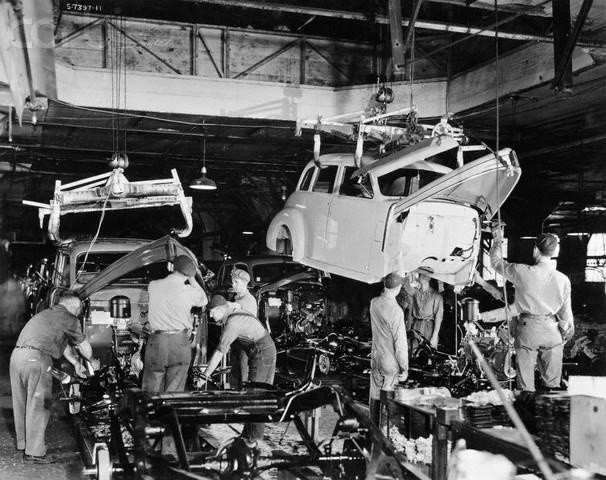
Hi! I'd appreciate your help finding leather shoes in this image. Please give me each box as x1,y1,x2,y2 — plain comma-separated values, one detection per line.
23,454,58,464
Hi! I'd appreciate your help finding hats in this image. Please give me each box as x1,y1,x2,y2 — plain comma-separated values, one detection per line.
175,255,196,277
205,295,228,309
536,232,557,251
385,273,401,289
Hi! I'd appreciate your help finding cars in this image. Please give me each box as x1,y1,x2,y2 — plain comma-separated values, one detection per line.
11,234,209,381
210,252,322,337
262,130,524,296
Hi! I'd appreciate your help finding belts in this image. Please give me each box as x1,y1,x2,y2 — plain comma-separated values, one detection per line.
520,313,555,318
155,330,188,334
16,346,39,351
414,318,433,322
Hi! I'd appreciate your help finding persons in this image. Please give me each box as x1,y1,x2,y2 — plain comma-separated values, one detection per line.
368,272,409,430
140,253,209,393
8,290,101,463
489,225,576,391
405,265,445,350
197,293,279,449
230,268,259,387
